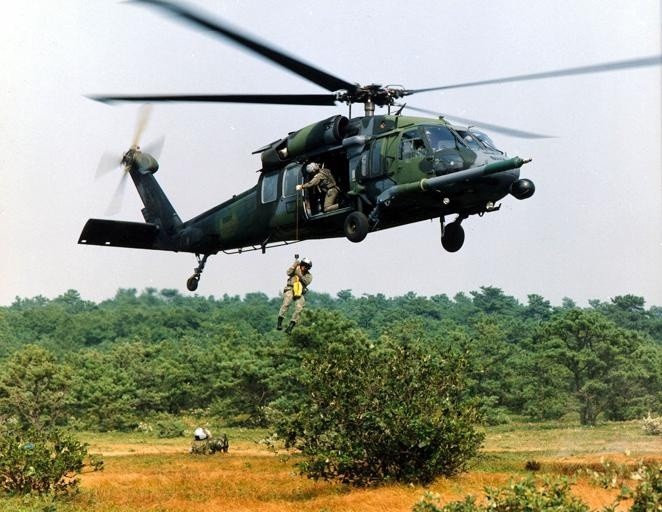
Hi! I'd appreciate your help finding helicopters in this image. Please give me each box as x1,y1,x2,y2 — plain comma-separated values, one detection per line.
78,1,662,292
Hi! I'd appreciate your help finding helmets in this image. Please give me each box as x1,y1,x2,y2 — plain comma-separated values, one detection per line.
300,257,312,269
306,162,319,173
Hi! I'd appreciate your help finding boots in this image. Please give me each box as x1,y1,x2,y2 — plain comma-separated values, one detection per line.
277,316,284,330
285,321,296,336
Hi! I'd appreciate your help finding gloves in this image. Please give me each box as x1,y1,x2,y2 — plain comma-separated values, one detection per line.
295,184,303,191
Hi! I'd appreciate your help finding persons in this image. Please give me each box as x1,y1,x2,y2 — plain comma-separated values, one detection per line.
277,257,314,336
296,162,345,212
